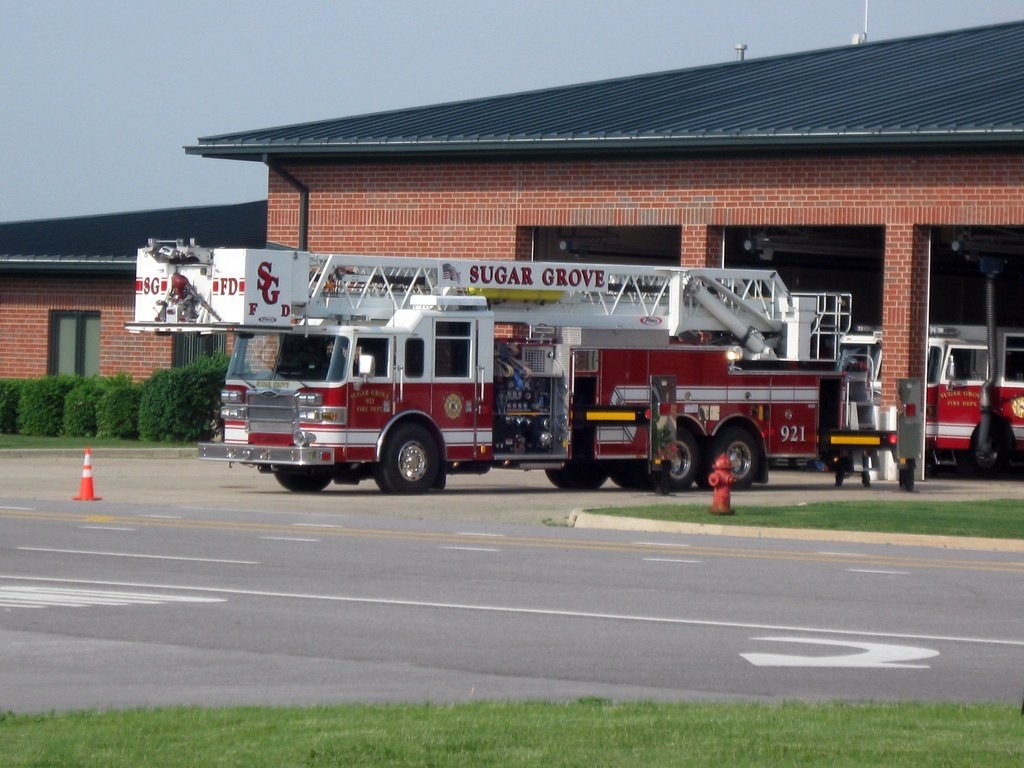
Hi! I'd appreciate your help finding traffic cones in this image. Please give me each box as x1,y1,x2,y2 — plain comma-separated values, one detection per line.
69,448,105,500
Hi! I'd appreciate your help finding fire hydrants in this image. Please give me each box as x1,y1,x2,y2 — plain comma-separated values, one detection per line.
708,450,737,514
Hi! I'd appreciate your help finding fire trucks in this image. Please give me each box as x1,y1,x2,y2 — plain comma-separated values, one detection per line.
833,323,1024,479
122,232,894,494
